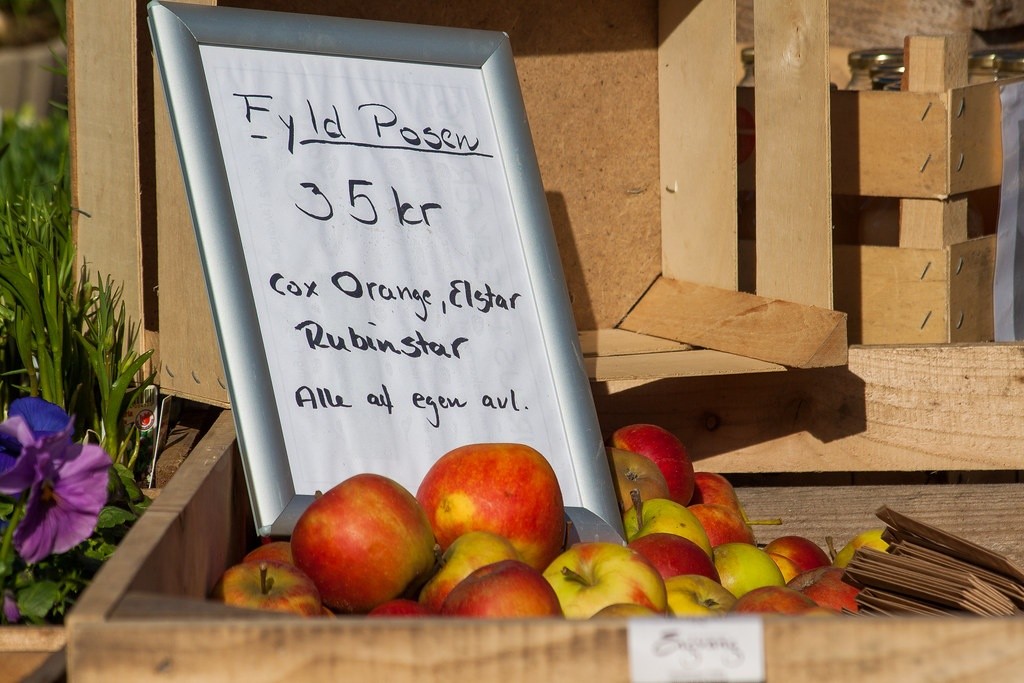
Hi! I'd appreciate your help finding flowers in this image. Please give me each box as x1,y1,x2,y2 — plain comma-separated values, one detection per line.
0,149,164,622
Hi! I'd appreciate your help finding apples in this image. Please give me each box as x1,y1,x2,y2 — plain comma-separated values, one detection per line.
205,424,893,618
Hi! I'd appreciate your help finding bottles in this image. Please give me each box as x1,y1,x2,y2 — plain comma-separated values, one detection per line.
739,46,1024,93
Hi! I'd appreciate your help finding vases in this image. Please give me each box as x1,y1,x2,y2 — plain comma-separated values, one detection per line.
0,382,239,683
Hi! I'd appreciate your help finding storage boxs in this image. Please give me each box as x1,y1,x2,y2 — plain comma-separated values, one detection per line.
64,341,1024,683
